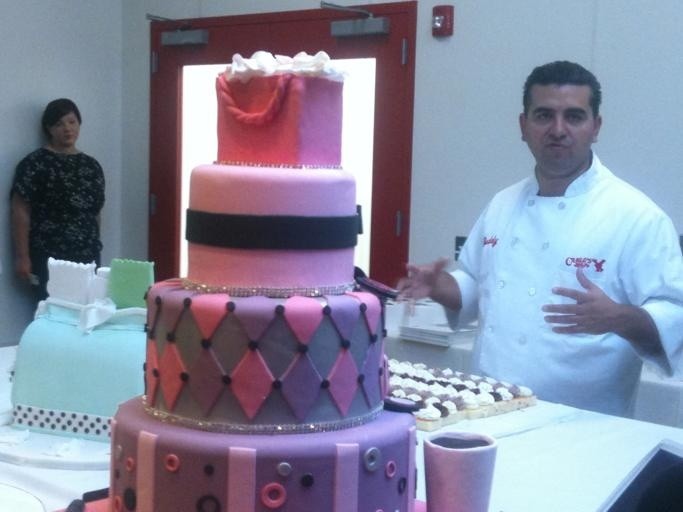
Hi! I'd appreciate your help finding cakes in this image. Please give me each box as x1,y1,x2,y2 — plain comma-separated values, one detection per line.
388,356,538,432
0,258,154,467
67,51,496,512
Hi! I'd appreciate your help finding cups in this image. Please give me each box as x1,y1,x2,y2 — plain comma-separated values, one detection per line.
423,432,497,511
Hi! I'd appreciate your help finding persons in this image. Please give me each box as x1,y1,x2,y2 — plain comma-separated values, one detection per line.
10,98,106,302
396,61,683,420
